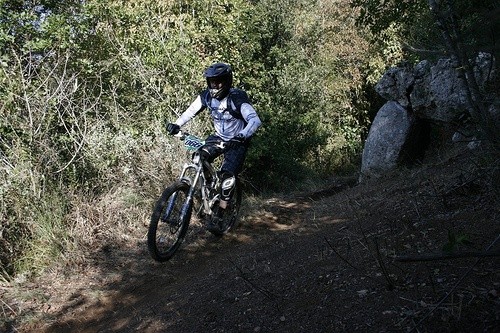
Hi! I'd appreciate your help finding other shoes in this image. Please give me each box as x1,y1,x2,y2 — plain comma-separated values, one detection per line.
206,216,224,231
197,183,210,198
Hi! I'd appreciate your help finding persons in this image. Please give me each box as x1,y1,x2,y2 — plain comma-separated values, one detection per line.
166,62,261,218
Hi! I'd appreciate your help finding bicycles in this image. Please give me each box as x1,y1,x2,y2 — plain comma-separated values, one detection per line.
147,124,243,263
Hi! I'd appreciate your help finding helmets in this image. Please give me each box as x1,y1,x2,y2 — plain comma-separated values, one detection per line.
202,63,233,98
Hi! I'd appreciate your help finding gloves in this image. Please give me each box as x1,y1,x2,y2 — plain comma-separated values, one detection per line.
165,123,180,136
228,135,243,150
